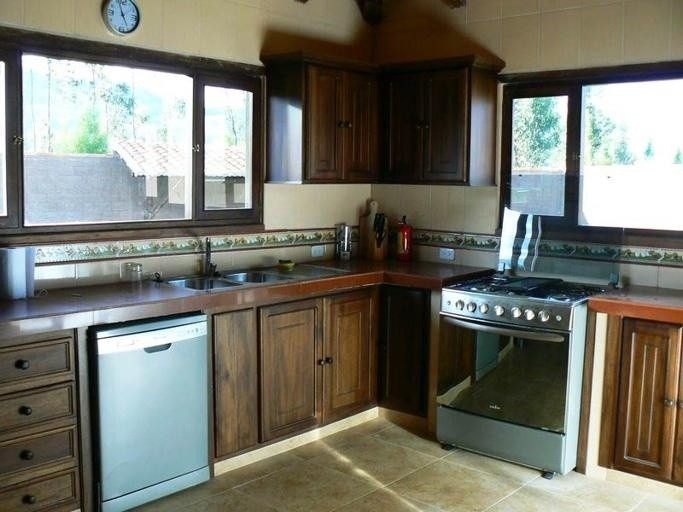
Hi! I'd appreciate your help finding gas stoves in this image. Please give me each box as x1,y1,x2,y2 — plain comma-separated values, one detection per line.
440,269,601,329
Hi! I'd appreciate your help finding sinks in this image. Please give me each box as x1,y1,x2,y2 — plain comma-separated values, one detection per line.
162,274,241,290
223,270,296,283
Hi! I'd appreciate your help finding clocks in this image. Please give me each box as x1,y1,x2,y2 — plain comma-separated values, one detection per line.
101,0,140,37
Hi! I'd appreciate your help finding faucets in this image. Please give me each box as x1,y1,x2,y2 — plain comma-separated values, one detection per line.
205,236,218,279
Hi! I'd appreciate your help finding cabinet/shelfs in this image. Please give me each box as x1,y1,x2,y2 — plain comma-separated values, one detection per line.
256,286,378,445
378,283,431,419
258,50,505,188
597,317,682,488
0,328,83,512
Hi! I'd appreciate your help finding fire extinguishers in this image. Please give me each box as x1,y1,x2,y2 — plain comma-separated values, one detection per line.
397,215,411,262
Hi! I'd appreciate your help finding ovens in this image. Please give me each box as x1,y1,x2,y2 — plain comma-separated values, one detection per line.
435,310,581,478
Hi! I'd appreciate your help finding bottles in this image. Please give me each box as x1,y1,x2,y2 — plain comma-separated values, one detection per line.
336,224,352,263
126,263,141,287
